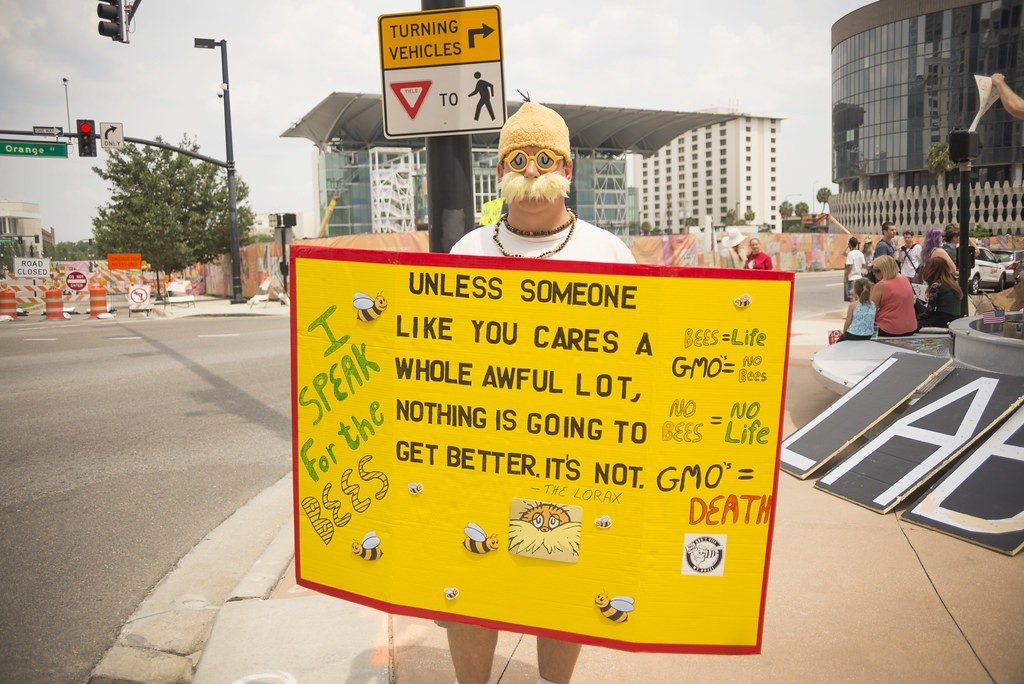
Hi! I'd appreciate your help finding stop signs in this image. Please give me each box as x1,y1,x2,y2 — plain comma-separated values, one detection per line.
65,272,87,290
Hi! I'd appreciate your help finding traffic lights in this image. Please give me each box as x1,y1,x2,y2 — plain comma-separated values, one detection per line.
195,38,248,303
283,214,296,227
269,214,281,228
77,120,96,157
97,0,127,43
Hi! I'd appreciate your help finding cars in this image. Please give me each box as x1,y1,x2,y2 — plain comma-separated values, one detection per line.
969,247,1006,294
989,249,1024,288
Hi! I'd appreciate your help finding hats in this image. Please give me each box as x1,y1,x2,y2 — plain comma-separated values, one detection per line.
497,89,570,163
722,229,747,247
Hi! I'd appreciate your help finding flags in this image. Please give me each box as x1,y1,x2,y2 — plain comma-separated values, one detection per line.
983,310,1005,324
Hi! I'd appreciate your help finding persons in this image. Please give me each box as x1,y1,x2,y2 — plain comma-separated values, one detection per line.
917,256,963,328
862,238,874,277
873,222,896,260
990,72,1024,120
836,279,876,342
870,255,918,336
921,228,956,274
893,229,922,284
435,102,637,684
941,223,960,268
744,238,773,270
844,237,866,319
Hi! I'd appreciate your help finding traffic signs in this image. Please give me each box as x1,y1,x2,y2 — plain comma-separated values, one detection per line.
33,126,64,137
378,4,506,140
101,122,124,149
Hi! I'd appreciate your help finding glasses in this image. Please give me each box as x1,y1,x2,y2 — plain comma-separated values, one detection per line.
504,149,564,172
873,269,880,273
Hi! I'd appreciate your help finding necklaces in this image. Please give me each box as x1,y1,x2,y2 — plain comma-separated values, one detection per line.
492,208,578,259
